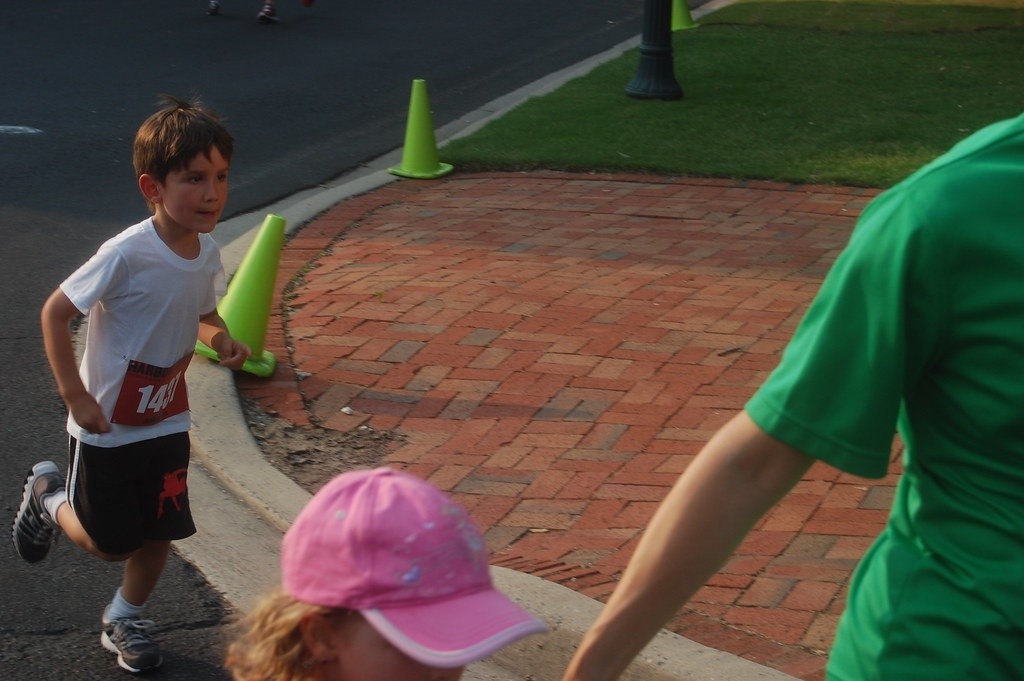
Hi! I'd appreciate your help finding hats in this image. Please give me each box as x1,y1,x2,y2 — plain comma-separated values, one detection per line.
282,465,548,668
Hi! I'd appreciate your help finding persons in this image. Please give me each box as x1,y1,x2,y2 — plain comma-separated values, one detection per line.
560,111,1024,681
12,94,252,672
226,466,549,681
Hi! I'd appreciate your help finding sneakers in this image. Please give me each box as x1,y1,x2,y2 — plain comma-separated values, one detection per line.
101,603,162,672
11,460,65,565
